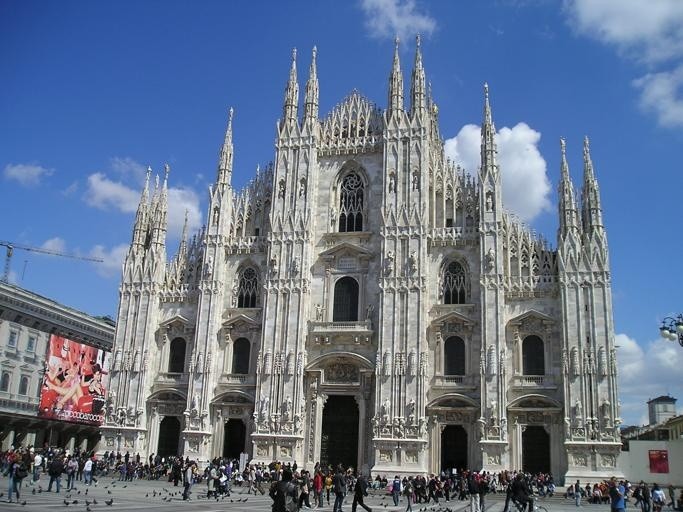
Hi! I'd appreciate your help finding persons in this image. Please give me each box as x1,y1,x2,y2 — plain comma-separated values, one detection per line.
43,363,107,413
562,476,683,512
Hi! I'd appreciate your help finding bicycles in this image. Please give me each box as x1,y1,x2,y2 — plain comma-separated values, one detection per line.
506,493,550,512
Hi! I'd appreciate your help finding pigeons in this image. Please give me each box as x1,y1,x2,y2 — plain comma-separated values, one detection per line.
0,481,127,512
343,494,467,512
145,488,249,503
